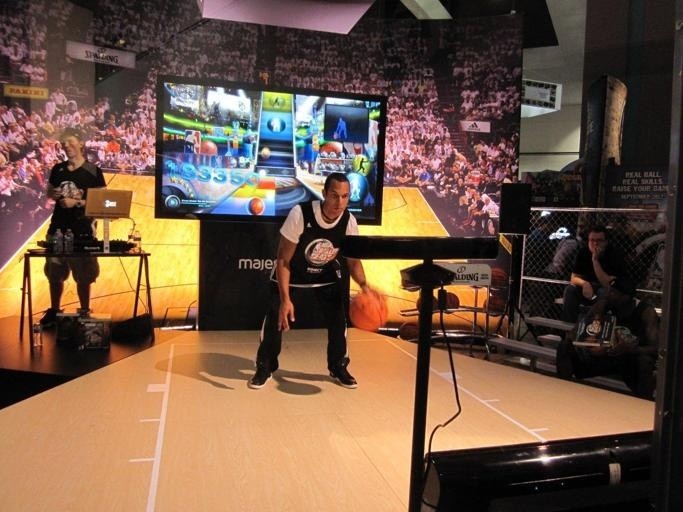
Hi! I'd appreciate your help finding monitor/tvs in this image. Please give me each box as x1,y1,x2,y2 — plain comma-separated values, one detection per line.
154,75,388,226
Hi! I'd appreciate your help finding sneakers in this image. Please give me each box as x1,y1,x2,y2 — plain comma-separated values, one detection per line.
39,308,63,327
247,368,273,390
328,366,359,390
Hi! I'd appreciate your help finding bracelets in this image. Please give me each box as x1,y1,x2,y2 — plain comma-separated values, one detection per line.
358,281,366,288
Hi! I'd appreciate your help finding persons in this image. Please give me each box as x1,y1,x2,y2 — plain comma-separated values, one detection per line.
518,208,662,402
39,127,107,326
1,0,526,239
246,171,387,389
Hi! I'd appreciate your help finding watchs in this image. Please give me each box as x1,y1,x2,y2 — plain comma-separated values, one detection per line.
75,198,80,208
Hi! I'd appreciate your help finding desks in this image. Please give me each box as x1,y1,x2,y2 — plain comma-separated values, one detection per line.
17,246,155,344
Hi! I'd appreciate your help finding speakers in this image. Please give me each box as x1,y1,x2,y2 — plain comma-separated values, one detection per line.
500,182,531,236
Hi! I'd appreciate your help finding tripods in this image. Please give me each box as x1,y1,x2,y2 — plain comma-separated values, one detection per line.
484,235,543,360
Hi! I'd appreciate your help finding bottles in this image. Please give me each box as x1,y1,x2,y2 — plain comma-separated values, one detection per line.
127,228,133,252
46,229,73,254
133,230,141,253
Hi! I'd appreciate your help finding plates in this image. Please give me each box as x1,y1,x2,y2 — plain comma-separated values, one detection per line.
26,248,48,254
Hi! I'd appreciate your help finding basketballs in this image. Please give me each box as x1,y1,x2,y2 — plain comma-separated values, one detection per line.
416,296,438,313
483,296,505,317
492,267,507,281
399,321,419,340
350,291,389,330
444,293,459,314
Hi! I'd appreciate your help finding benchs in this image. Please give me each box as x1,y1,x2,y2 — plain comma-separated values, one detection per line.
485,294,662,399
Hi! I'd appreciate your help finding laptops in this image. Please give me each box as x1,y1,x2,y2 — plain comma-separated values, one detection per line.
85,188,133,218
